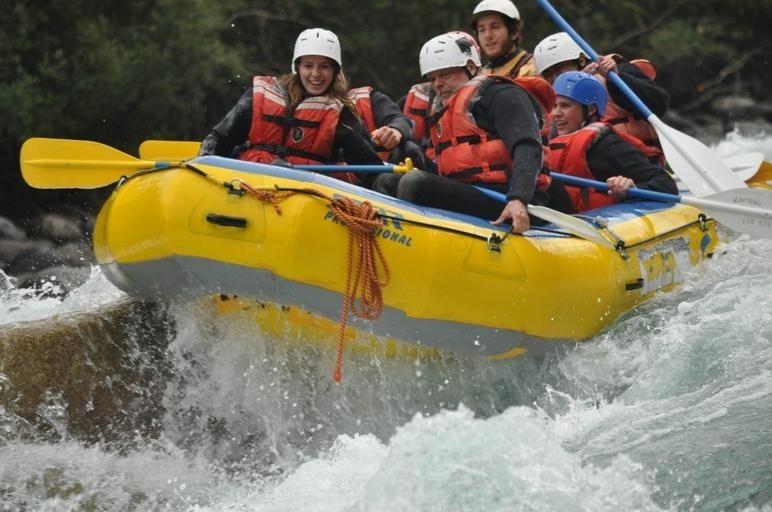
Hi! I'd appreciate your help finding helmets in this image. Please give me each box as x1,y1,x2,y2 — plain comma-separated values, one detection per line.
471,0,521,24
533,32,592,77
291,27,342,73
418,32,481,78
552,70,608,118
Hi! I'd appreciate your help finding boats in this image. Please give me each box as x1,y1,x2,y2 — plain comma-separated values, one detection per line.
91,162,772,359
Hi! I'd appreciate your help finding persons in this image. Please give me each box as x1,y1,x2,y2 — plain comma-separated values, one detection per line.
197,29,388,188
323,0,678,234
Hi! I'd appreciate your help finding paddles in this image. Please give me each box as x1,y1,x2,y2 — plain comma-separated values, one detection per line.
472,185,609,246
552,173,772,219
666,152,763,192
19,137,414,190
539,0,749,198
135,140,204,162
